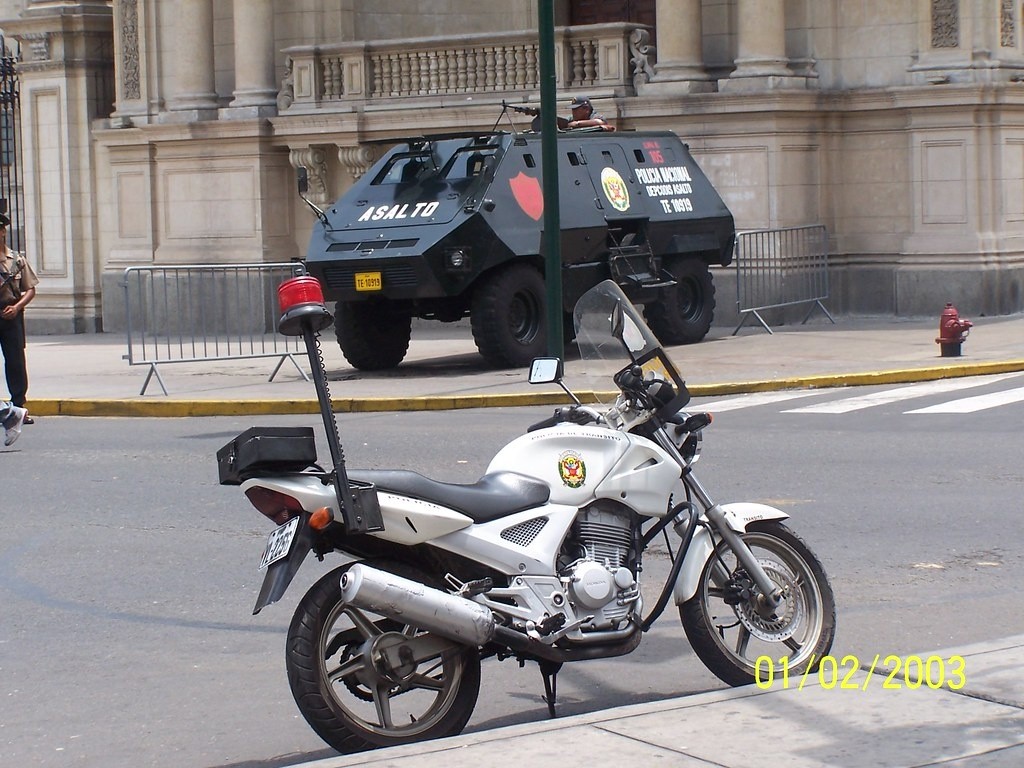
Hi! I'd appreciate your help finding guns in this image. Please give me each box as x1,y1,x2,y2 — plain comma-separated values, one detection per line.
493,100,568,136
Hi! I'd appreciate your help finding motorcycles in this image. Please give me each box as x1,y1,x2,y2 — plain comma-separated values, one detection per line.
216,264,837,757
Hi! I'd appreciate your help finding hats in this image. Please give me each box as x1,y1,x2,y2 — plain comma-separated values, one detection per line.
0,212,10,228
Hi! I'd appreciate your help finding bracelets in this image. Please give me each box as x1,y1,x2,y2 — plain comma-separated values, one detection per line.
576,120,579,128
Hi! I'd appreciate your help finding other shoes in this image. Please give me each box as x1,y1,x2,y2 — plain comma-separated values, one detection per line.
25,417,34,425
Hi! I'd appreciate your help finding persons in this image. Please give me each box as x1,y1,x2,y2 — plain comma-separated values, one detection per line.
0,212,40,424
0,399,28,447
567,95,607,129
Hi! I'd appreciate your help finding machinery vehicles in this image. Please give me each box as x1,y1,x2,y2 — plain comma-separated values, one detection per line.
295,100,736,368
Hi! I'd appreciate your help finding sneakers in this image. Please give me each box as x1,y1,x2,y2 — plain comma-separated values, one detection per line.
5,407,28,446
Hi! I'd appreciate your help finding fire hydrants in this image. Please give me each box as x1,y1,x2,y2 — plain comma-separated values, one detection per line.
935,302,973,358
